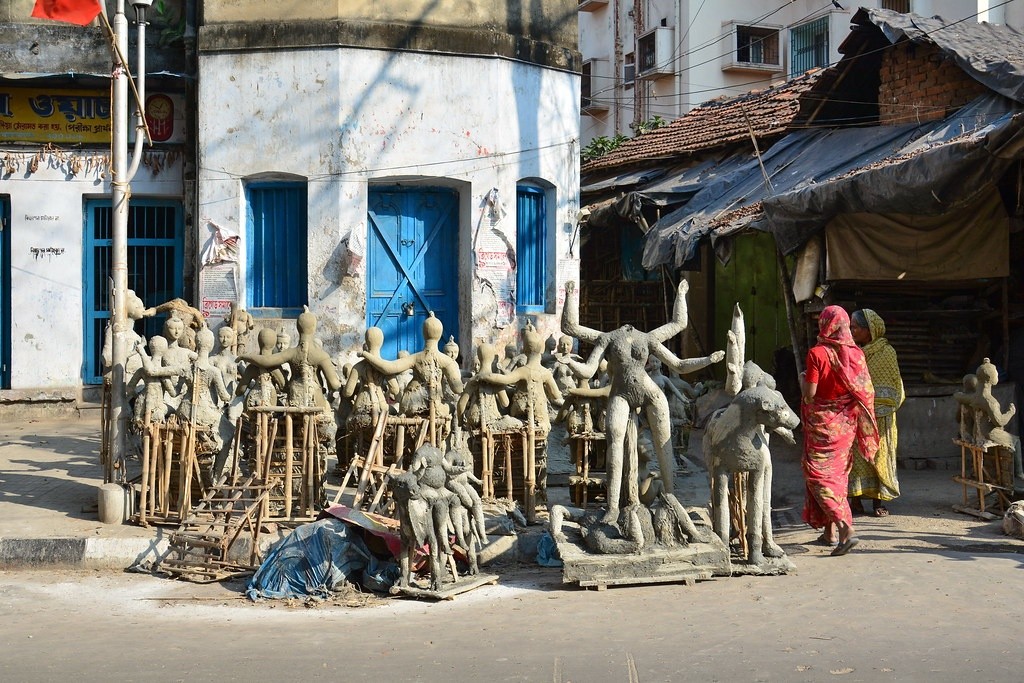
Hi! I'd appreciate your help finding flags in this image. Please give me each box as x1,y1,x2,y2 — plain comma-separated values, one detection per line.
31,0,102,29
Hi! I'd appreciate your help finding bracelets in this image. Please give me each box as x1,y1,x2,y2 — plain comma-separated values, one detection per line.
799,372,806,376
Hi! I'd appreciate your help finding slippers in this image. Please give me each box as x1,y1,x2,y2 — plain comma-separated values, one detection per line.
817,534,840,546
831,537,859,556
850,506,865,514
872,506,889,516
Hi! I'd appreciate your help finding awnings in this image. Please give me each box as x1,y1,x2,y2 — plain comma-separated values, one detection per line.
581,145,769,352
641,91,1023,373
581,165,678,194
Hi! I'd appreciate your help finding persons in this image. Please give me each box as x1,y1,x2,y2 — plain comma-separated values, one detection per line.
798,305,880,555
953,359,1024,480
98,285,800,565
847,309,906,517
